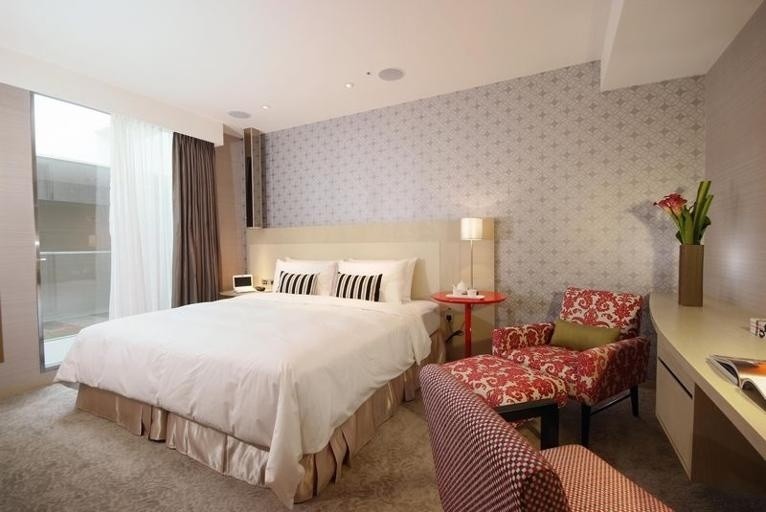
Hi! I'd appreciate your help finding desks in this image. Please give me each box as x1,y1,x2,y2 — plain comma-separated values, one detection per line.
431,290,507,358
648,294,766,482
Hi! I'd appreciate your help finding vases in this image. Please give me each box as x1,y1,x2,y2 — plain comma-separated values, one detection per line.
678,245,704,307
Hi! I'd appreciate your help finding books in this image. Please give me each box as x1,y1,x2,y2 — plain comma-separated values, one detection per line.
750,318,766,340
706,354,766,402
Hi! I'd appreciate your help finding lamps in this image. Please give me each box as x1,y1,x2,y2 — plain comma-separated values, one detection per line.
461,217,483,290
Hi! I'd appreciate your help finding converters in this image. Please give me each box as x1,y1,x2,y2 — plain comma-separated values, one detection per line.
447,315,451,320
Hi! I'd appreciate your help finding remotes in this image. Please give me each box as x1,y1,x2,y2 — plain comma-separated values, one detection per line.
255,287,265,291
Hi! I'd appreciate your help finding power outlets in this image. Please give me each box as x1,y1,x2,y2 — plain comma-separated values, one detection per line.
444,311,453,322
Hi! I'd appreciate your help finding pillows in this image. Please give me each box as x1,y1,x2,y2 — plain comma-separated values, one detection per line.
549,320,620,351
271,256,418,304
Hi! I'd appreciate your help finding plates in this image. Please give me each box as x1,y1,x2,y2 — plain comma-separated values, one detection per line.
446,294,485,299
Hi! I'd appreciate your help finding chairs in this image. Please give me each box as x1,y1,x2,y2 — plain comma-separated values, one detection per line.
491,288,652,449
419,364,673,512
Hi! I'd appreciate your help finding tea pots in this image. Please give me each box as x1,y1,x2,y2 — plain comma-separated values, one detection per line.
454,281,467,295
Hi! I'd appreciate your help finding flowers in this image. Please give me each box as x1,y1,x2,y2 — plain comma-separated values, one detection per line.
653,181,713,245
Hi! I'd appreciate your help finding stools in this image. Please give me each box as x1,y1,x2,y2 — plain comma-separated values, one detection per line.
441,353,569,450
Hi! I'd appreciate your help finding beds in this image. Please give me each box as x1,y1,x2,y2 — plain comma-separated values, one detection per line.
54,217,496,511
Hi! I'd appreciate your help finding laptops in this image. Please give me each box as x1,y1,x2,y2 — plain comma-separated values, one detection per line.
232,274,257,293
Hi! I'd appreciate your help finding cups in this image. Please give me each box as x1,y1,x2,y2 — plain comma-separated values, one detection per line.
467,290,478,297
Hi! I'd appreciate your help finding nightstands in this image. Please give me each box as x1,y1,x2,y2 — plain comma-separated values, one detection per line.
220,291,238,297
